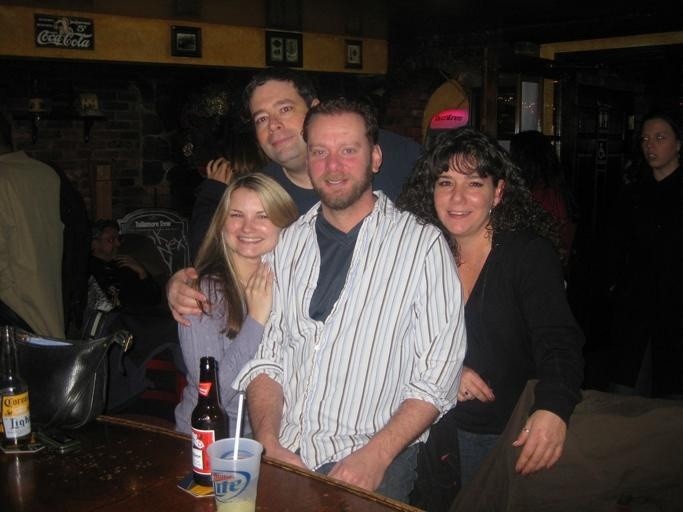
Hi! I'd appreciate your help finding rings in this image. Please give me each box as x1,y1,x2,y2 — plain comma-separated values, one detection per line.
521,429,530,432
463,390,469,396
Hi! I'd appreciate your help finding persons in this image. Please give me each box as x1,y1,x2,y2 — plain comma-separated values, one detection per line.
0,114,64,339
396,127,584,508
185,140,261,260
230,96,468,505
509,130,569,289
86,215,189,375
608,115,682,397
166,68,420,327
174,171,300,441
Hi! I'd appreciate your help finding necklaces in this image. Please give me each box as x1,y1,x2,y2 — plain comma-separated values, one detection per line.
458,249,491,272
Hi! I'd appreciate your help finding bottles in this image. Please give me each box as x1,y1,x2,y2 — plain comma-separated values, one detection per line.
0,324,33,456
190,356,230,489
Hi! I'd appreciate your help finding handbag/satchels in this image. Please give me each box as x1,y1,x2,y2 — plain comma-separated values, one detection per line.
14,324,136,454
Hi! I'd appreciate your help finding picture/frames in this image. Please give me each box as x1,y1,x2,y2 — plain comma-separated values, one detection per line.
266,29,304,69
343,39,362,69
35,13,96,51
170,23,203,58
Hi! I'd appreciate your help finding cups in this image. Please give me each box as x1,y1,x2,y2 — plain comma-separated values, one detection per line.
206,437,265,511
